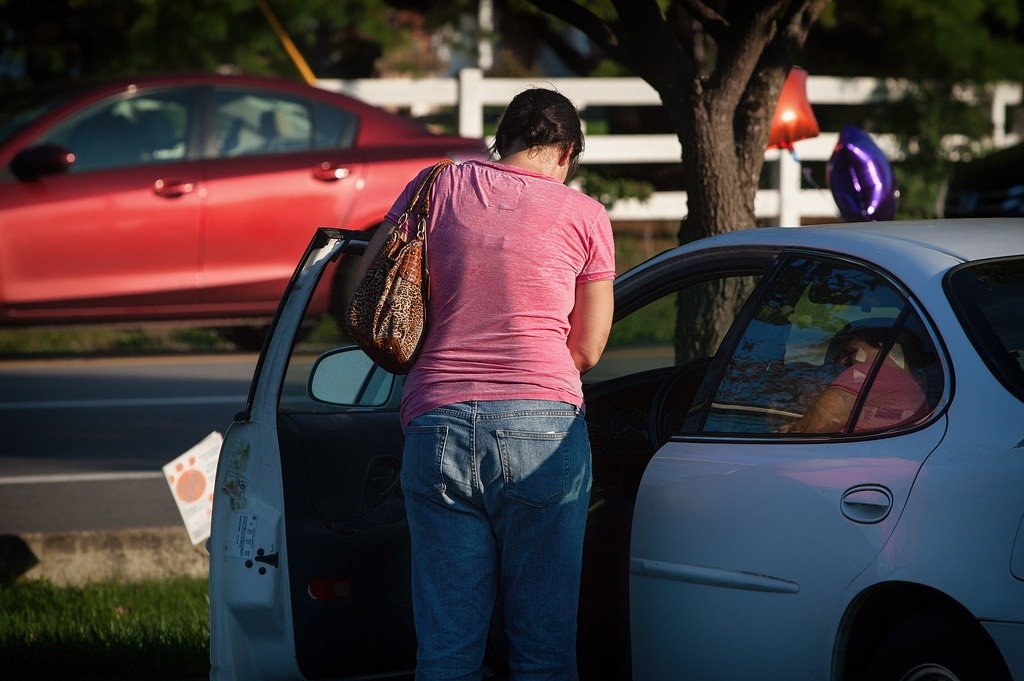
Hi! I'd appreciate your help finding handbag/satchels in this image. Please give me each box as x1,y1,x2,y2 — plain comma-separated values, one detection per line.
347,158,456,373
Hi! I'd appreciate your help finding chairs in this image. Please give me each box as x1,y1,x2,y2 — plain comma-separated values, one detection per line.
217,110,282,157
713,316,895,428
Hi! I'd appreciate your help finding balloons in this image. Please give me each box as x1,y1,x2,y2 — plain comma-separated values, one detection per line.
825,125,894,221
766,66,820,152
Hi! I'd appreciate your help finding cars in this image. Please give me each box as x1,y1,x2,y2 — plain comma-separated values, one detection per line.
208,223,1021,679
1,71,489,351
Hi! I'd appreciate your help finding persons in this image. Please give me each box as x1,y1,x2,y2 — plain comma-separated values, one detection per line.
776,329,931,433
344,89,615,680
